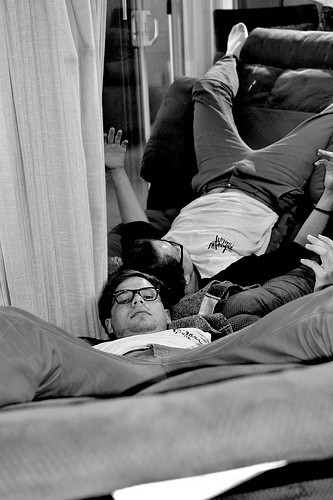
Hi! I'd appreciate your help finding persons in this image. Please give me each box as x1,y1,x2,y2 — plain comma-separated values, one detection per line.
0,234,333,406
103,22,333,288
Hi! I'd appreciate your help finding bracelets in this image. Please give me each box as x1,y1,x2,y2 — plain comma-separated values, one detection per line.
313,204,331,215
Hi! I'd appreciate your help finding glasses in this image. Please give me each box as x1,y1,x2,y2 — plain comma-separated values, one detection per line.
111,285,160,304
156,238,184,268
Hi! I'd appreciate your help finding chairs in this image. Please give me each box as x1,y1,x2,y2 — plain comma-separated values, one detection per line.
105,27,333,318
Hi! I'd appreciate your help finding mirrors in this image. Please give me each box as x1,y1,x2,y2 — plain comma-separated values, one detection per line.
102,0,333,326
